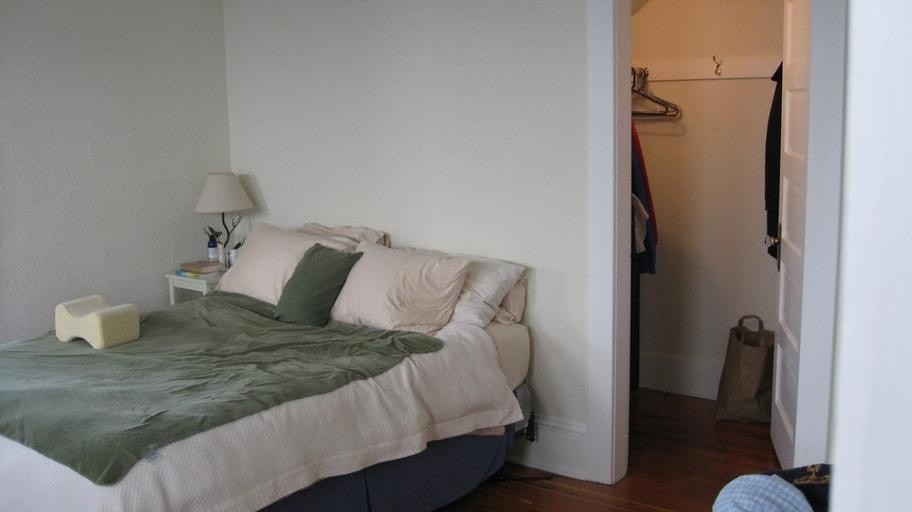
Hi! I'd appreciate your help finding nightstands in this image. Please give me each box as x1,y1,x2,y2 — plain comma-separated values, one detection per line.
164,270,226,305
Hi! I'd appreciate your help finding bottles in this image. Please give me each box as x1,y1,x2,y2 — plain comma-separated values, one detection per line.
223,249,229,269
217,240,225,267
229,249,237,267
206,233,218,261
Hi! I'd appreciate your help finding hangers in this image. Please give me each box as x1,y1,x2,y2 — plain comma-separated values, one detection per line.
630,65,680,120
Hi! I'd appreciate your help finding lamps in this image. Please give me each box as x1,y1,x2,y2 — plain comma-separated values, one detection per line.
192,171,257,269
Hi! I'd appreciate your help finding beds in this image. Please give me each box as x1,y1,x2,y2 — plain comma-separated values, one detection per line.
0,289,536,511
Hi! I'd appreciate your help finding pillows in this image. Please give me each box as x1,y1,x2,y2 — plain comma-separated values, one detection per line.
329,239,471,334
271,241,365,330
214,222,356,306
392,247,527,326
298,223,389,249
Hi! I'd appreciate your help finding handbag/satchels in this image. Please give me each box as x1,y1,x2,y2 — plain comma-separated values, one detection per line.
715,325,774,426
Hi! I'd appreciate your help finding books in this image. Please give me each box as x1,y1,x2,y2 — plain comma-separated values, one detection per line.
174,259,227,279
